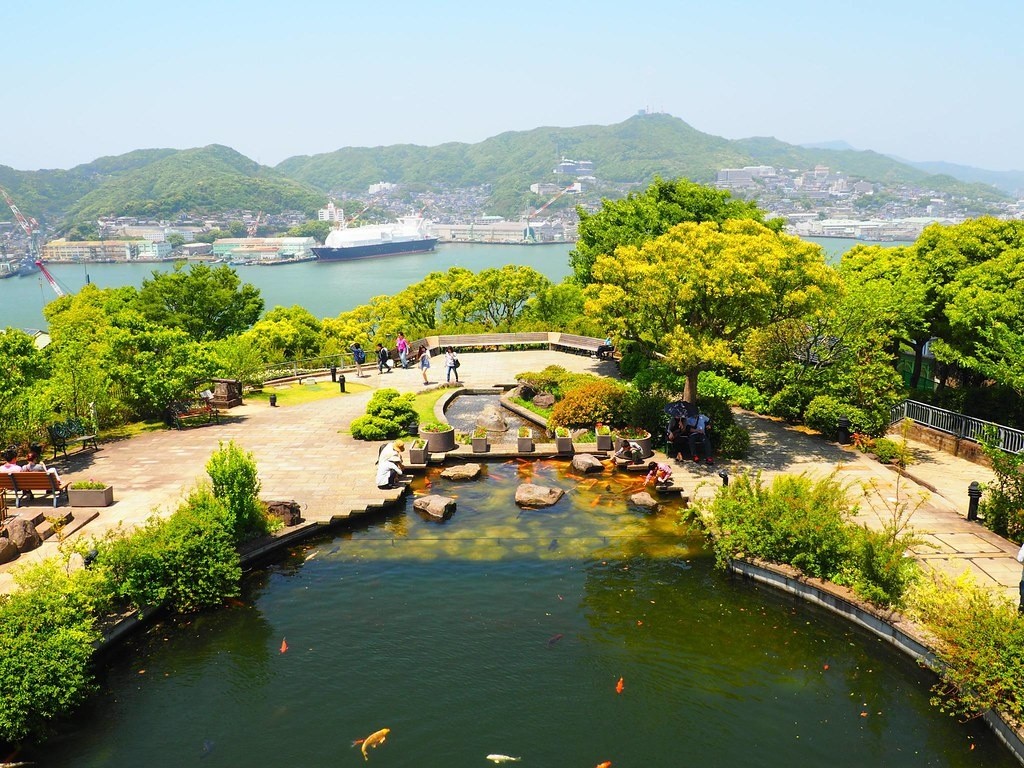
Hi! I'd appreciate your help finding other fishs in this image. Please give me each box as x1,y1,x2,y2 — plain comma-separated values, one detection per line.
587,469,655,507
547,635,562,645
279,637,288,653
327,546,341,556
412,477,462,498
306,549,319,561
521,507,538,511
230,598,244,607
590,495,601,506
351,728,390,761
486,754,522,764
566,473,583,481
201,741,218,760
0,751,36,768
548,539,558,552
615,675,624,693
488,474,503,480
501,458,566,484
596,761,611,768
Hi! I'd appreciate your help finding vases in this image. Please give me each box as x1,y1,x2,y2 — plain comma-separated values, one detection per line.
68,485,113,507
614,431,652,459
595,426,611,450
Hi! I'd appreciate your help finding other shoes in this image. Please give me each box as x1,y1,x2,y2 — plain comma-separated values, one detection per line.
378,371,382,374
403,366,405,369
628,461,634,465
456,379,458,382
355,373,359,376
424,381,428,385
387,367,391,372
361,375,364,377
678,454,684,462
46,490,52,494
676,455,679,461
693,455,699,462
406,364,407,369
707,457,713,463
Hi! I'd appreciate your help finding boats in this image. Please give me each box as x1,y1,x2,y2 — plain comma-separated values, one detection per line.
310,206,442,262
19,255,45,278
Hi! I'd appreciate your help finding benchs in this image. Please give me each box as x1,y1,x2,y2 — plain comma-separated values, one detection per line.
169,398,219,430
46,417,98,460
661,420,713,454
409,332,617,361
389,346,418,367
0,472,72,507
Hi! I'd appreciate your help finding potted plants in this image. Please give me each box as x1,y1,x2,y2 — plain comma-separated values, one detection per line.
555,426,571,452
419,422,454,452
472,426,488,452
409,438,428,463
517,425,532,452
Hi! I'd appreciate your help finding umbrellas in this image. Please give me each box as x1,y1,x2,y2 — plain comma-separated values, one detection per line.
664,400,698,417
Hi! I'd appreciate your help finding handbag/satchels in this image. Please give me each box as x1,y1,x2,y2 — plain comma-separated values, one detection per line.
454,359,460,368
681,425,697,436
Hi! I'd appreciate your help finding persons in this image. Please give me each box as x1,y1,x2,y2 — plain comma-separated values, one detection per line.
396,332,410,369
22,451,61,494
350,343,365,377
443,346,458,382
0,450,26,494
641,461,672,487
418,345,431,385
667,414,714,463
376,439,405,489
591,336,614,358
374,343,391,375
1017,543,1024,619
611,440,643,465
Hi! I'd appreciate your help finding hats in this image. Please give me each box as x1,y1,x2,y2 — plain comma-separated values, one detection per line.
393,439,405,452
388,456,401,461
687,408,698,416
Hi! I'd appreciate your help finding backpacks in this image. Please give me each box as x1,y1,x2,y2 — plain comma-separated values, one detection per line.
357,349,365,364
388,350,391,359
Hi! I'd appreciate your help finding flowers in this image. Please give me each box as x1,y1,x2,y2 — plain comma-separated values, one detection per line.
72,478,108,490
596,422,609,435
616,424,648,440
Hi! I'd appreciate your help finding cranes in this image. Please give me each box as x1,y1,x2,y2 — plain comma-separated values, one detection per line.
523,180,574,239
35,260,66,296
0,188,42,256
247,211,263,237
